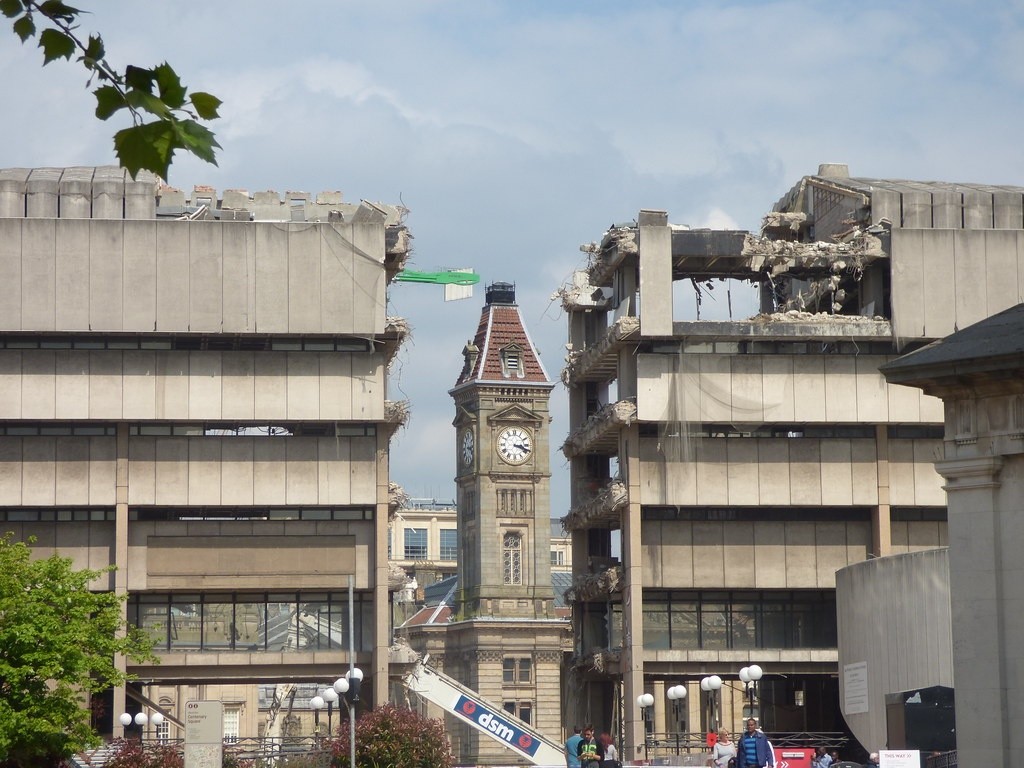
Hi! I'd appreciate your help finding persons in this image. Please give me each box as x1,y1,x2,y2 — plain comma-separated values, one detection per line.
564,725,619,768
829,750,842,768
736,719,778,768
811,746,833,768
864,753,879,768
713,727,737,768
707,729,717,751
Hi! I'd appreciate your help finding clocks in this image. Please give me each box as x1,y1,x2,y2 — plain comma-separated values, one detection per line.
497,426,533,463
462,432,473,465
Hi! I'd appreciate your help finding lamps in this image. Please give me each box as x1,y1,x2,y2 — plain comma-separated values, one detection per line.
196,197,213,206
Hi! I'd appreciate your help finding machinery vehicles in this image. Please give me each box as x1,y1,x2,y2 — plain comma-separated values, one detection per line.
258,612,573,768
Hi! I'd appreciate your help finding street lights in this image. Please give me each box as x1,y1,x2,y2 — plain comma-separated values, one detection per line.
151,713,163,744
334,667,364,768
309,696,324,749
700,674,721,749
120,713,148,744
667,684,687,757
323,688,339,737
638,693,653,761
739,665,763,720
